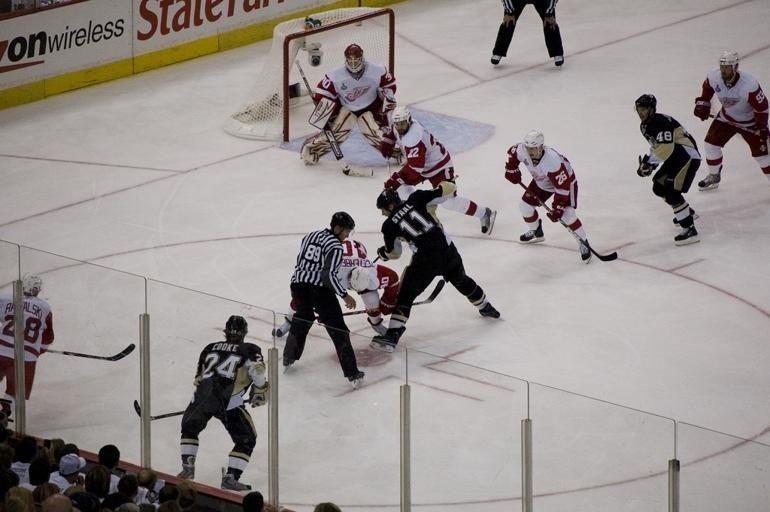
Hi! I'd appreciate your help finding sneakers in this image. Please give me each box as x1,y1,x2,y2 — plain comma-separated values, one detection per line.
277,317,296,364
349,370,364,382
491,55,502,64
673,167,723,241
479,304,500,318
177,456,195,479
481,208,491,233
520,219,543,241
221,467,251,490
580,239,590,260
555,56,564,66
367,318,406,345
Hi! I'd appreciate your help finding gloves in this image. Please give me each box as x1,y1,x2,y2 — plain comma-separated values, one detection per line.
378,246,389,261
694,97,711,120
379,293,397,315
384,172,405,190
547,201,566,222
505,162,521,184
637,154,659,177
381,133,397,157
249,382,269,408
754,126,769,139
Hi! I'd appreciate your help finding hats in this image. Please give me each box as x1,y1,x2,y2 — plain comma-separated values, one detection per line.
59,453,86,475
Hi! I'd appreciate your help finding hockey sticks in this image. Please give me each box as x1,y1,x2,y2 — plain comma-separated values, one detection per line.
294,60,373,176
133,399,252,420
342,280,444,315
519,181,618,262
45,344,135,361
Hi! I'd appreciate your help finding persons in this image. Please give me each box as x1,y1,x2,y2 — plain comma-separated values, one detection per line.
381,103,492,234
631,92,702,243
490,0,565,68
691,46,770,191
177,314,272,492
504,127,590,262
0,413,340,511
274,211,401,383
299,42,400,163
372,175,499,352
0,271,55,429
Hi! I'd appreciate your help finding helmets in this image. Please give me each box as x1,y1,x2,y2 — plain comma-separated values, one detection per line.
21,273,41,294
351,267,371,291
377,189,400,209
524,129,544,147
392,107,412,126
226,316,247,337
635,94,656,106
344,44,363,73
331,212,354,228
719,51,739,66
